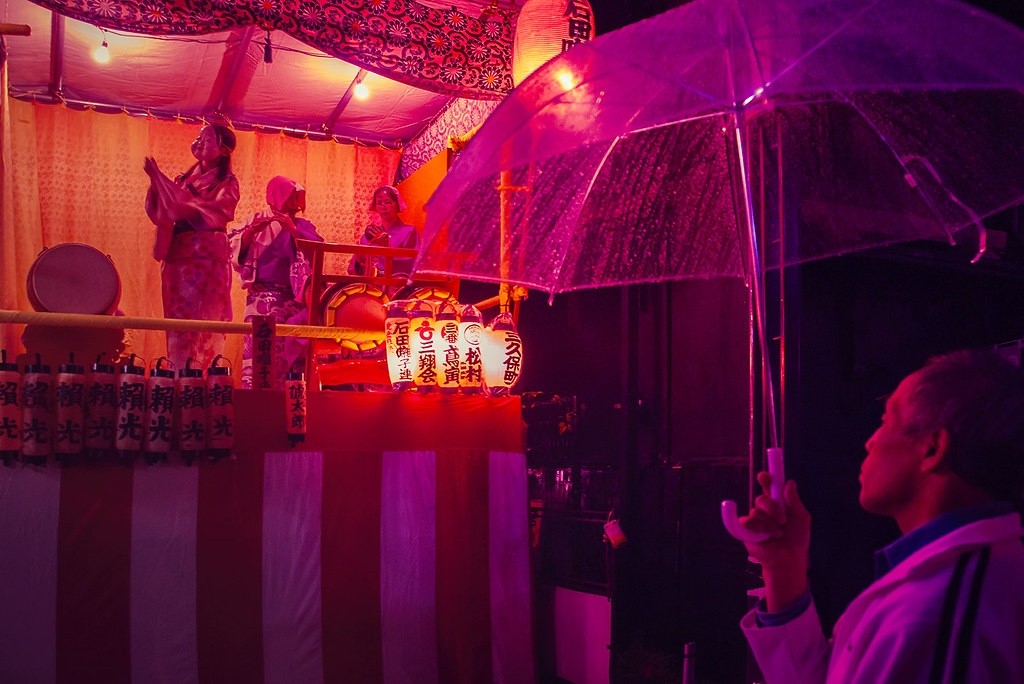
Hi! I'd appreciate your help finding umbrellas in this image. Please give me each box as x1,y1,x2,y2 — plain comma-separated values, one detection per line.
410,0,1023,544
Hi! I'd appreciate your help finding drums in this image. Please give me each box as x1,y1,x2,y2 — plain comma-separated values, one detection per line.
25,241,122,315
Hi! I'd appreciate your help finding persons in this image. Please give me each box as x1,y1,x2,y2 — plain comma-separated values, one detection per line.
144,124,241,366
346,184,418,295
229,176,326,390
739,350,1024,684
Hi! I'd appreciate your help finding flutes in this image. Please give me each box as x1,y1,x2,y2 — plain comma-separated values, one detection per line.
226,207,301,240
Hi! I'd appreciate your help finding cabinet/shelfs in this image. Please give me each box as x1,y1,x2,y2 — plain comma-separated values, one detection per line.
541,508,695,684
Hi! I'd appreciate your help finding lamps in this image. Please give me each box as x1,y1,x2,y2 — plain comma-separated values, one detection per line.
263,28,272,63
